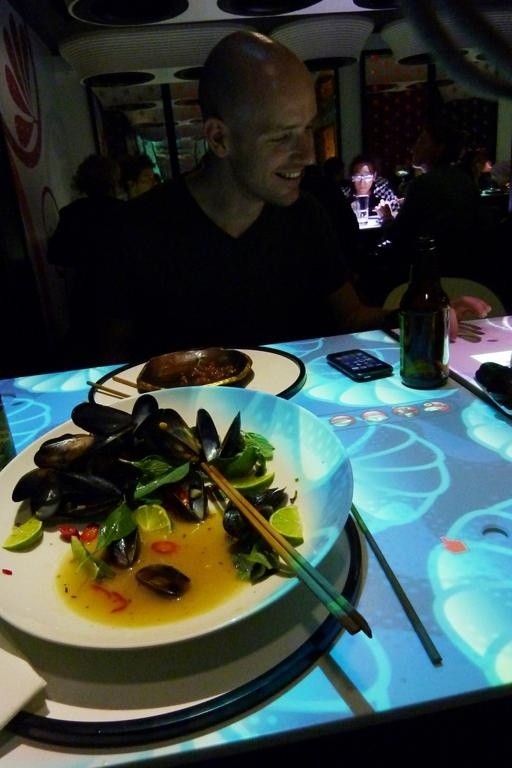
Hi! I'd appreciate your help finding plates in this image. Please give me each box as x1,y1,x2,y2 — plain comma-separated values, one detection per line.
0,514,360,745
2,384,354,651
89,345,304,403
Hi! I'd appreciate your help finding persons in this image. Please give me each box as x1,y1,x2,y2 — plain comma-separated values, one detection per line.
82,28,496,344
300,120,511,314
44,144,167,321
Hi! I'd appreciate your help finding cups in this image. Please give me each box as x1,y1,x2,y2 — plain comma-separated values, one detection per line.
356,196,369,217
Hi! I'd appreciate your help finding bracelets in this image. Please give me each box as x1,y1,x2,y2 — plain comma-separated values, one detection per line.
378,308,401,325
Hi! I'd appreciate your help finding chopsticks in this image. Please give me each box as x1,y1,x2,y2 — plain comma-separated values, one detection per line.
202,460,370,635
349,505,443,665
87,378,138,397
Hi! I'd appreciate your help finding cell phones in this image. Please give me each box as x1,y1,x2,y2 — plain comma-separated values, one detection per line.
326,349,393,382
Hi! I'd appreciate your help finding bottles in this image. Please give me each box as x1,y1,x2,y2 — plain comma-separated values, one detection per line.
398,235,451,390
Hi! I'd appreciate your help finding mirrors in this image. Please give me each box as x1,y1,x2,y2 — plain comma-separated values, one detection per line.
86,67,342,203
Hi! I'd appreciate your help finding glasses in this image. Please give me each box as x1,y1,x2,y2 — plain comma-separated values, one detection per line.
352,175,373,182
